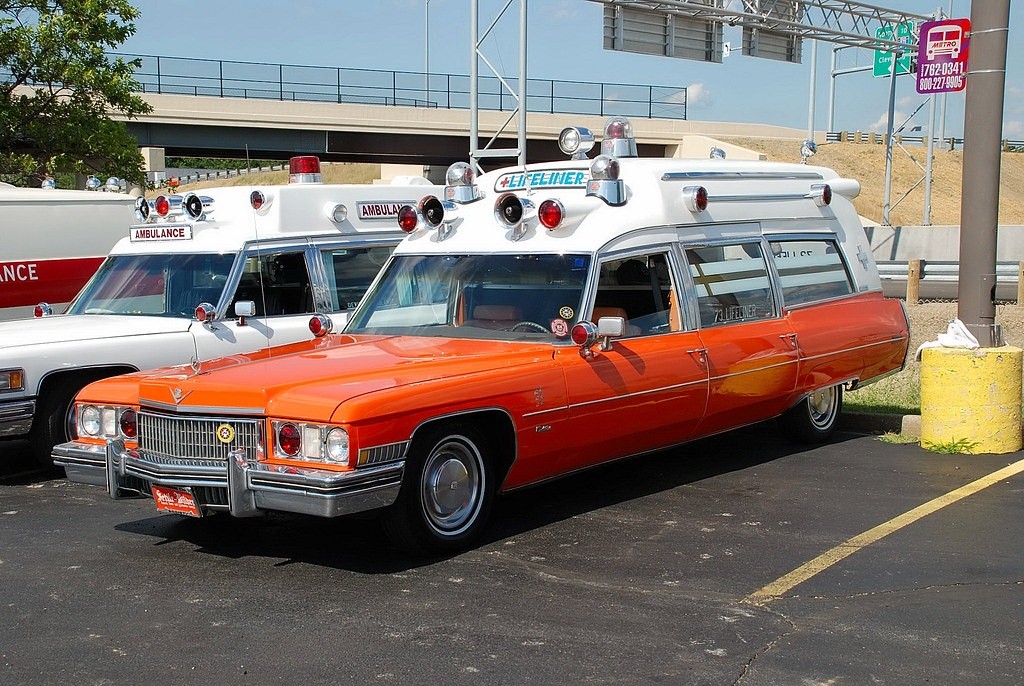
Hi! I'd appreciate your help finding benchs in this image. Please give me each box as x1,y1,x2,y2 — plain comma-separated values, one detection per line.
179,287,289,315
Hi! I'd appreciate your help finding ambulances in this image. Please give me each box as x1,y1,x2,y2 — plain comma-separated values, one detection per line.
0,154,467,453
53,117,912,545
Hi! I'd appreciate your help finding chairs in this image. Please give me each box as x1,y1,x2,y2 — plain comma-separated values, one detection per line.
591,307,641,336
460,304,528,336
548,269,578,285
616,258,650,284
